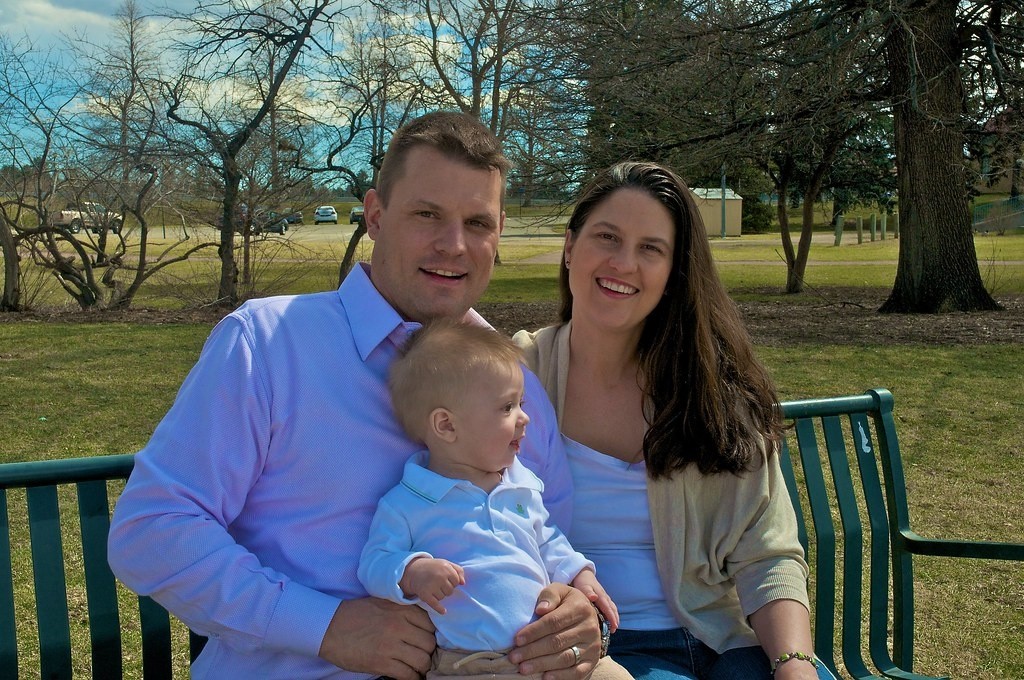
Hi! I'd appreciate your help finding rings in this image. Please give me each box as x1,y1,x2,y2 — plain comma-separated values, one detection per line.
570,646,580,665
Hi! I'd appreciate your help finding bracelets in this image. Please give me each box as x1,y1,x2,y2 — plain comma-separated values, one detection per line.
770,652,820,678
590,601,611,659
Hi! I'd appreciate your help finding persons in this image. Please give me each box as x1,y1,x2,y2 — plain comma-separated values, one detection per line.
358,310,640,680
106,107,575,680
507,160,843,680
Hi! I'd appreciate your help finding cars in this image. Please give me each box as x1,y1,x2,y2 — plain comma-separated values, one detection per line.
313,205,338,225
282,208,304,224
348,205,364,224
213,202,290,237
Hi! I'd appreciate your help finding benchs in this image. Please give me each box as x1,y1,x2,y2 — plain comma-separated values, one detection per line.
0,386,1024,680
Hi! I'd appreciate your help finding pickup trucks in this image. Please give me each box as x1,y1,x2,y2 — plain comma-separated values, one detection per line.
39,201,123,234
